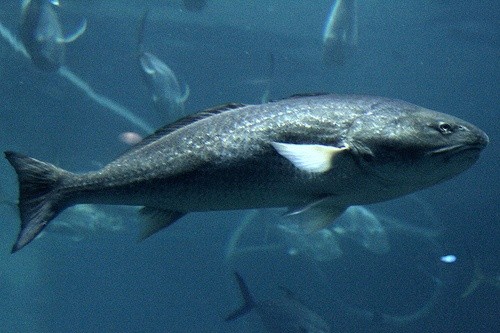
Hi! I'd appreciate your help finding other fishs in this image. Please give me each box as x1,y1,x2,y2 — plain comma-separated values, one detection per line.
18,0,87,73
321,0,359,70
225,271,331,333
136,51,191,127
332,205,390,256
275,224,343,262
3,93,490,255
